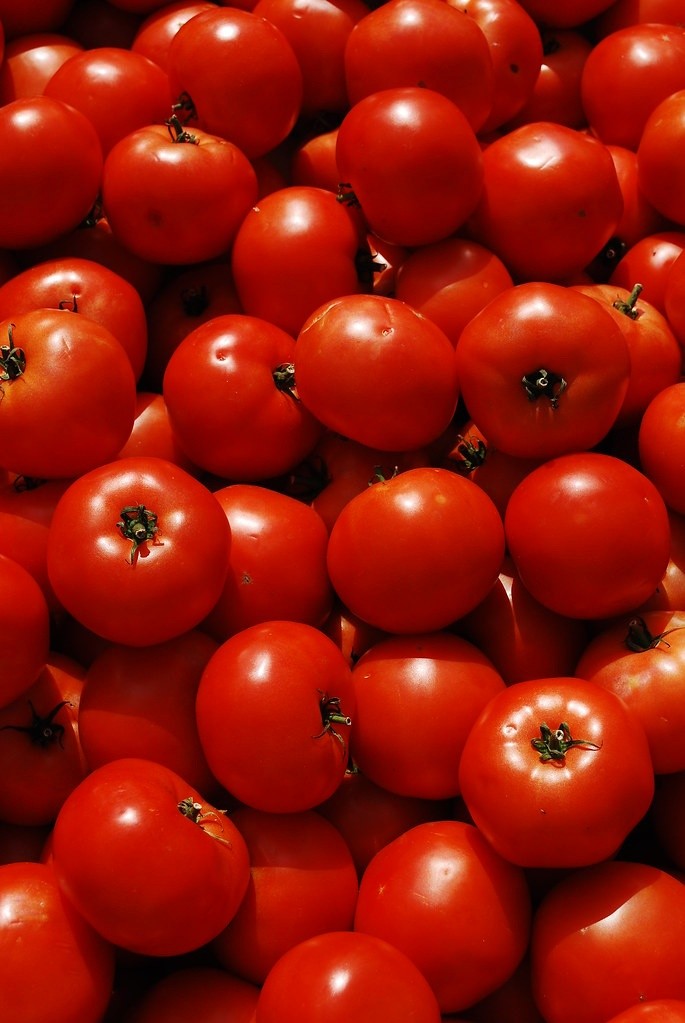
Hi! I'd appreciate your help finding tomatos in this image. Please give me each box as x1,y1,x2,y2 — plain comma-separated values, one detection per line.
0,0,685,1023
